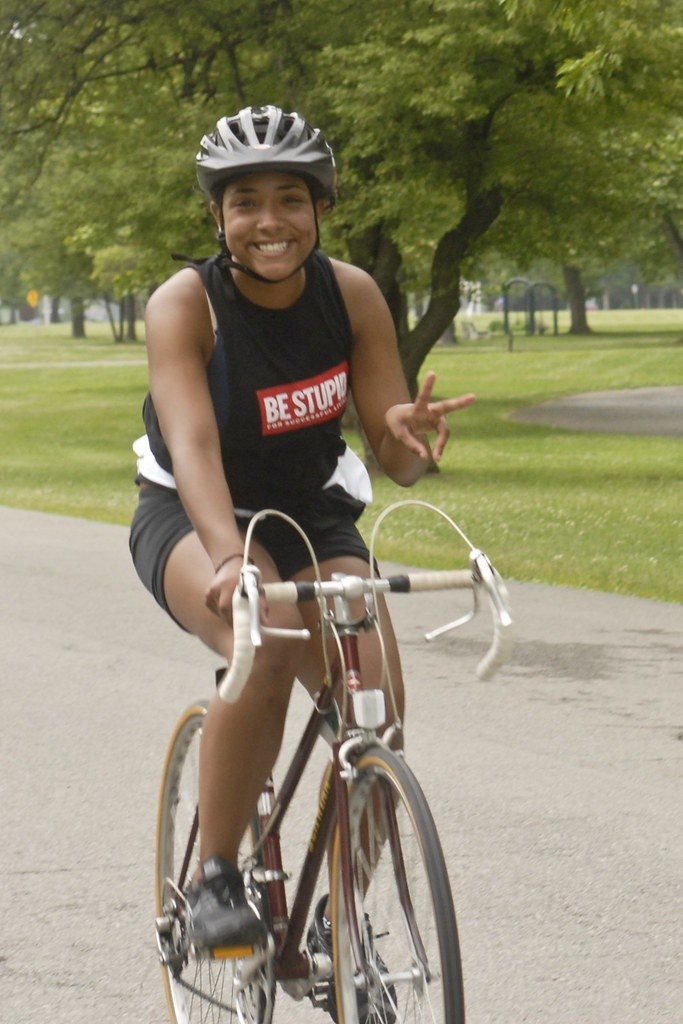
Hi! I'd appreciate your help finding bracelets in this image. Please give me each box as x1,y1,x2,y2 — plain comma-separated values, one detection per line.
217,552,253,574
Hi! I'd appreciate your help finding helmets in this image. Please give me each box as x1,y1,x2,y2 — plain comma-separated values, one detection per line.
195,104,337,208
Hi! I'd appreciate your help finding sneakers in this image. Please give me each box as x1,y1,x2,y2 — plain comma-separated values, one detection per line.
182,855,266,954
307,893,397,1024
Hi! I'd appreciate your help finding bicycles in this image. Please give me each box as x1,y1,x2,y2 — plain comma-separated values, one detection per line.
151,497,520,1024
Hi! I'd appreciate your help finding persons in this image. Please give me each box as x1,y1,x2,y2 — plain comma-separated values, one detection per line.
131,102,478,1024
